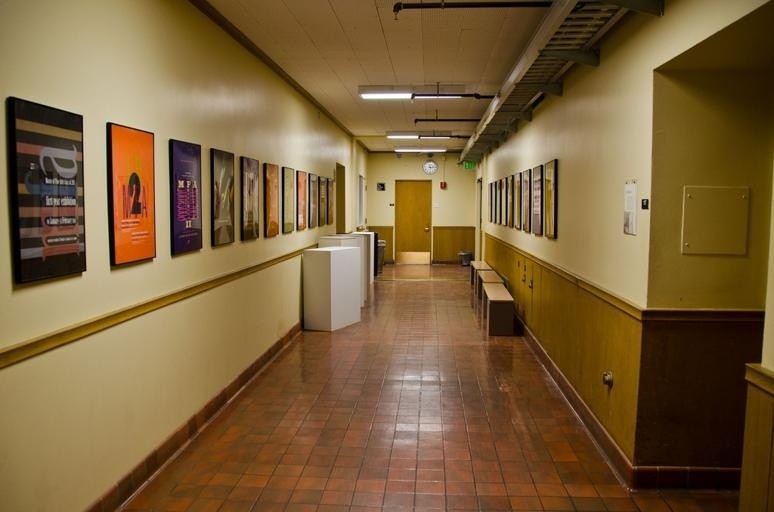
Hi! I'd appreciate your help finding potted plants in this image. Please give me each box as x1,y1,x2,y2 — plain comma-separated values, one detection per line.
486,158,559,240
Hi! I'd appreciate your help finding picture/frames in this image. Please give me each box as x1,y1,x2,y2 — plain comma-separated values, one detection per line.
239,154,261,242
168,138,203,258
261,162,279,239
281,167,297,233
6,95,85,282
209,148,236,247
106,122,155,266
296,173,332,231
486,158,559,240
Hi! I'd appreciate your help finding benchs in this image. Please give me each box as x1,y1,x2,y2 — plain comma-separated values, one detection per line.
469,260,515,336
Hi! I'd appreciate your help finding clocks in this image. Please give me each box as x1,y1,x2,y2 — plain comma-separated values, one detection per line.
422,160,438,176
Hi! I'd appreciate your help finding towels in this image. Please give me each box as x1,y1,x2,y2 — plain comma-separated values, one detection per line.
456,251,473,266
377,240,387,273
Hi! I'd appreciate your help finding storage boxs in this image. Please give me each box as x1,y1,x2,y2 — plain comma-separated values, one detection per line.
469,260,515,336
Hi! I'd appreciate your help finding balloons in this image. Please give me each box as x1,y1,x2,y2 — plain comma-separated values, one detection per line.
422,160,438,176
